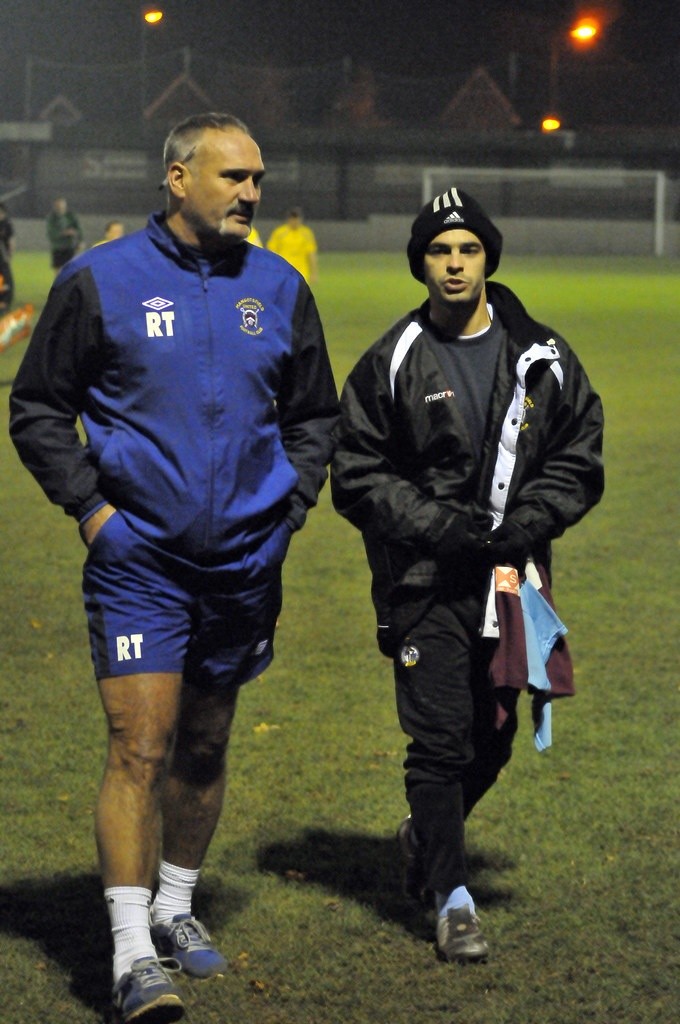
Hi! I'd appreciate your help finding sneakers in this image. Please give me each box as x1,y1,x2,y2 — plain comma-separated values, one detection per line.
110,957,186,1024
148,905,229,981
398,819,429,897
433,905,489,958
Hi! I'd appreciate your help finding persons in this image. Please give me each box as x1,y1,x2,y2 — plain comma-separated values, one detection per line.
264,203,321,290
329,184,605,964
91,221,126,250
47,197,86,275
0,201,17,317
9,111,345,1024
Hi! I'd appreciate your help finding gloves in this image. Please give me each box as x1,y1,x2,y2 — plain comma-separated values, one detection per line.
436,518,486,573
481,528,532,576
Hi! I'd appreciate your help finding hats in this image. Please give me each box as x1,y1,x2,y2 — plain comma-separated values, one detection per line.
406,188,504,289
